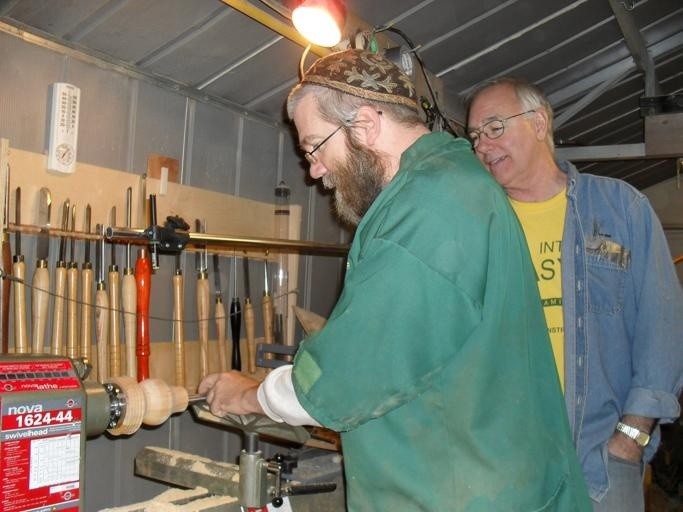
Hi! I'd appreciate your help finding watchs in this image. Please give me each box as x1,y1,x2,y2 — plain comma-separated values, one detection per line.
616,421,650,447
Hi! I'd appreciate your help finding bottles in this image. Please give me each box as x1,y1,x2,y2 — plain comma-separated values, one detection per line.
269,181,291,360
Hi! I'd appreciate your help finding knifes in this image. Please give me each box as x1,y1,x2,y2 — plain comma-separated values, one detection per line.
194,217,211,379
30,187,52,354
243,251,256,374
213,252,229,374
94,223,109,384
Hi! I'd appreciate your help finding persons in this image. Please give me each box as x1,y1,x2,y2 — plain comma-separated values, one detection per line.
465,77,683,512
196,49,593,512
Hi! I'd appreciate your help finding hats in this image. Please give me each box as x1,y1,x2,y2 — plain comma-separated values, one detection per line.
303,49,417,108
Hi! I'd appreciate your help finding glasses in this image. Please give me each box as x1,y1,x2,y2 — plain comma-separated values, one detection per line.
305,111,383,164
465,109,534,146
